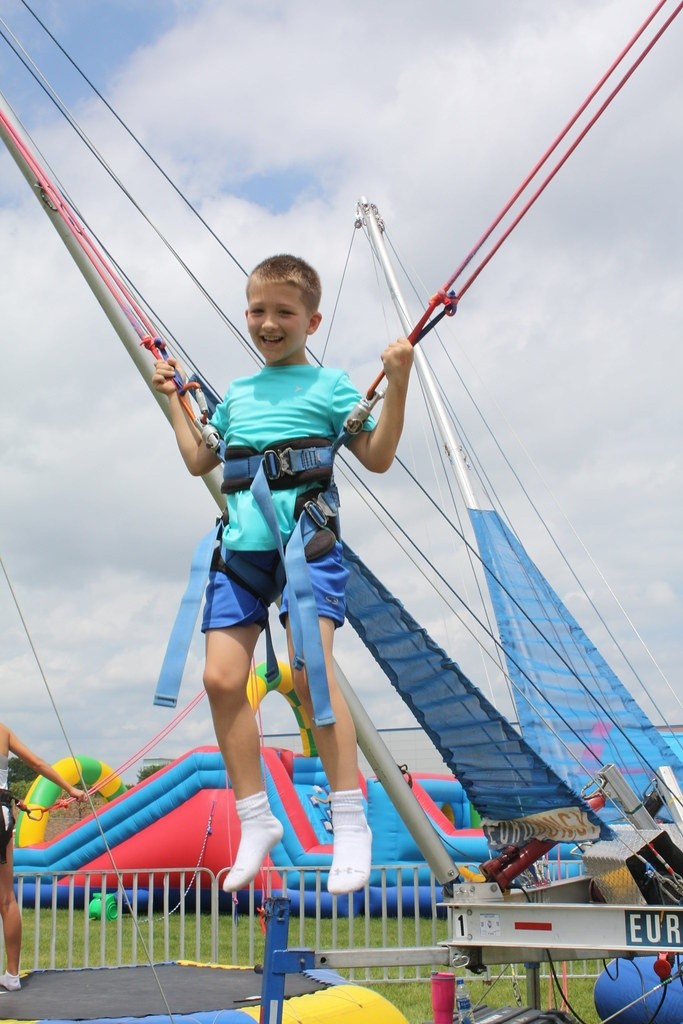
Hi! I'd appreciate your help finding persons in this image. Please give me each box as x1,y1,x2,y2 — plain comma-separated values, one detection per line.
149,254,417,894
0,722,88,992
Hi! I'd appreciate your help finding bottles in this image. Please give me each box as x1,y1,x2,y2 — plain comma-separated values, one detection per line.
455,979,475,1024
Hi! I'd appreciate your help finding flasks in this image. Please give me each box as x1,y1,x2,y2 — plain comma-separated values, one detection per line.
431,971,455,1024
